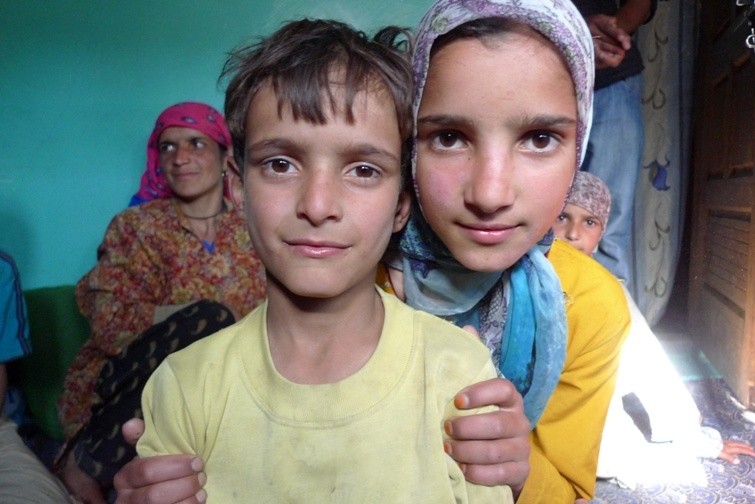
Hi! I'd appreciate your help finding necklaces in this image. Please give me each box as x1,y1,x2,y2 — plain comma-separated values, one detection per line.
182,209,224,221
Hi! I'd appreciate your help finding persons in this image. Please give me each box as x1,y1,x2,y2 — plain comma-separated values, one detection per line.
0,250,72,504
550,169,755,494
113,0,631,504
49,102,267,504
569,0,658,295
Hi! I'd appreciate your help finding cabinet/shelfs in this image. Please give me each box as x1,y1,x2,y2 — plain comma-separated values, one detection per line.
676,0,755,411
